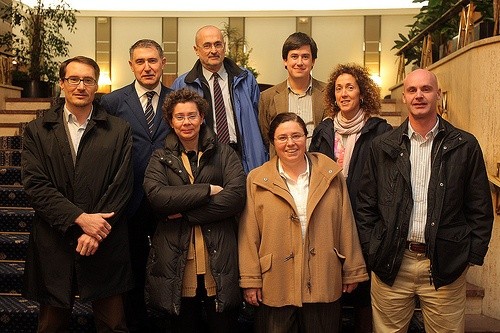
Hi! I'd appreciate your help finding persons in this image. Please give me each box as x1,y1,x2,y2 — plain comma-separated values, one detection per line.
142,87,248,333
309,63,394,333
101,39,174,333
169,25,269,322
354,69,493,333
258,32,332,161
20,56,132,333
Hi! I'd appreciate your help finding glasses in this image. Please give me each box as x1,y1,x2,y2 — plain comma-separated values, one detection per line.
170,115,201,124
63,75,99,86
273,133,306,144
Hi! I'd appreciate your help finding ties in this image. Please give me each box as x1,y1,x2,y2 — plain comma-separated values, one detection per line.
212,72,232,145
143,91,157,134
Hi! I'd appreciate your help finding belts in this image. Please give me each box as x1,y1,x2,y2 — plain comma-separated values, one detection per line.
404,241,427,254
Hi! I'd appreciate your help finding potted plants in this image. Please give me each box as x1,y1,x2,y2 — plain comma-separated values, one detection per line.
1,0,77,99
